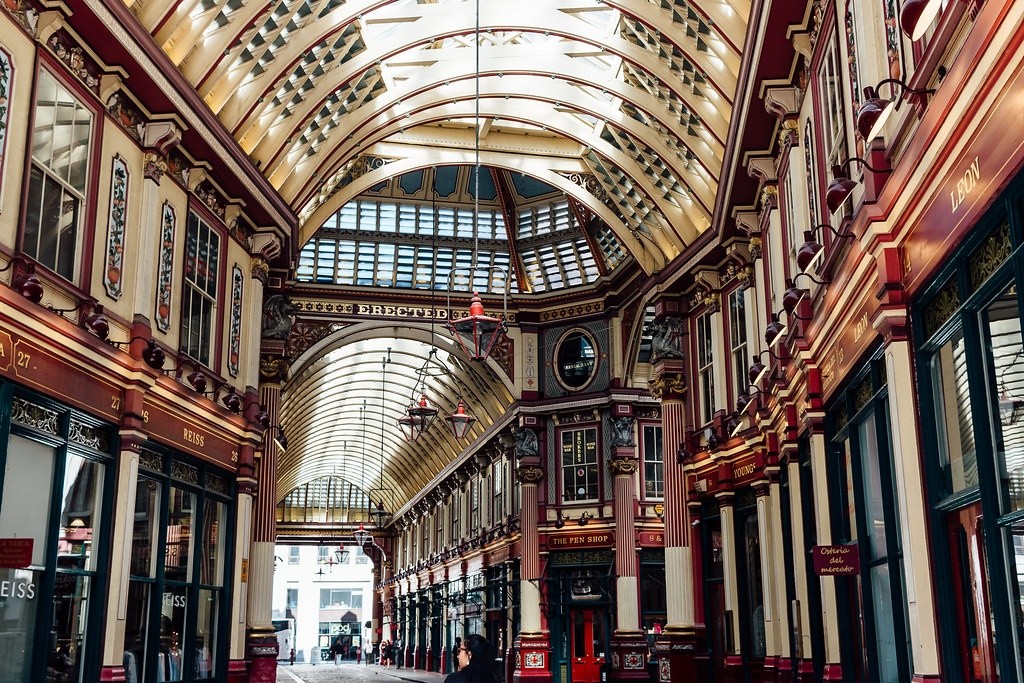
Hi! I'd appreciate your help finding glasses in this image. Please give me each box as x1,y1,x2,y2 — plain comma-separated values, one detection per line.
457,648,466,654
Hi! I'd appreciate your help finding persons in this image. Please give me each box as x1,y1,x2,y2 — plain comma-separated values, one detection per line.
382,635,405,670
364,638,373,666
451,637,461,673
330,640,346,669
290,649,294,665
444,634,502,683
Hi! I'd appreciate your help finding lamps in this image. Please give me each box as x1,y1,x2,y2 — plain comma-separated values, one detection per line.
737,385,772,415
783,273,831,317
109,337,165,370
261,423,288,453
0,256,44,304
204,383,240,410
51,299,109,341
728,411,776,436
395,166,476,442
857,78,937,144
797,224,856,274
164,360,207,393
765,309,812,347
237,402,270,428
900,0,941,42
749,350,795,385
441,0,509,363
826,158,893,216
